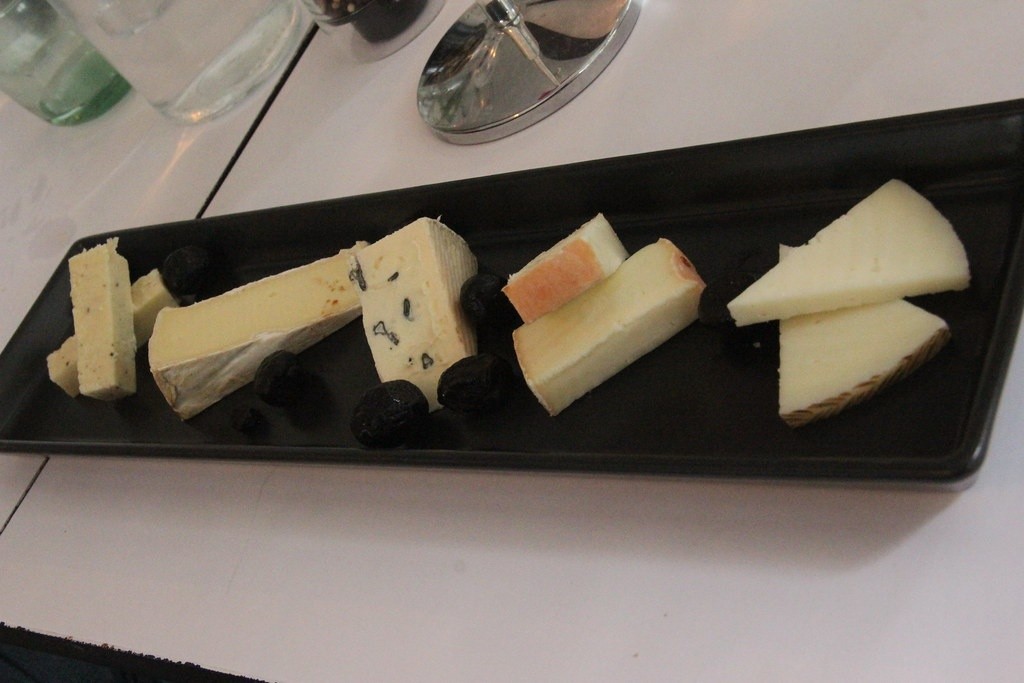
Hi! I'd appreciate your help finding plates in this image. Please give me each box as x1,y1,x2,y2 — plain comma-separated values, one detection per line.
0,96,1024,480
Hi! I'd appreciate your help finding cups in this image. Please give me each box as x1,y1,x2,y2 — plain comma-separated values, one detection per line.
46,0,310,126
0,0,137,125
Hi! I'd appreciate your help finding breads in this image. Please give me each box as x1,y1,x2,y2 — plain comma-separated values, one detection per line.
46,240,180,397
150,214,477,426
728,180,971,429
499,211,707,417
